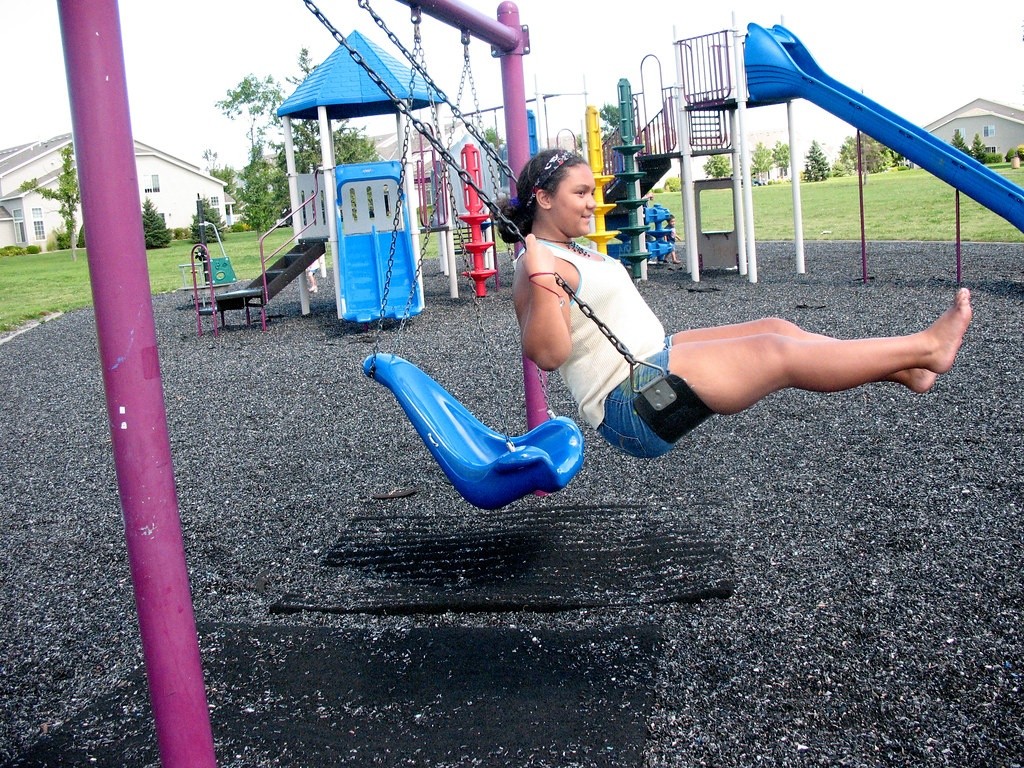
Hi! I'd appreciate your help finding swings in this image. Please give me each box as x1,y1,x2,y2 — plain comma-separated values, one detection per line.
303,0,718,444
361,20,586,511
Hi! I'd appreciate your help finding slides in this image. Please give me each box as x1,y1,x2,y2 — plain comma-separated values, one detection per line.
745,21,1023,244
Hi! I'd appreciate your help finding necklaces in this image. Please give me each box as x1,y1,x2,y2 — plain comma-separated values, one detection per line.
532,235,592,259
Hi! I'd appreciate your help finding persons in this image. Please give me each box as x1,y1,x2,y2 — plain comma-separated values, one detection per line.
661,215,682,265
751,172,767,187
484,146,972,459
